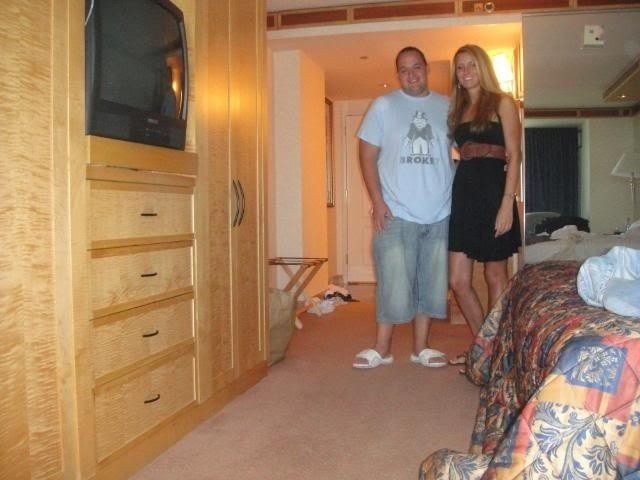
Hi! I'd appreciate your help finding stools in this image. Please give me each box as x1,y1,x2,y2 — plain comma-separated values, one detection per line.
269,256,329,332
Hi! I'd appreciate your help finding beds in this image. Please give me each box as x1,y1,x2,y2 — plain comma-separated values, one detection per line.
502,259,640,479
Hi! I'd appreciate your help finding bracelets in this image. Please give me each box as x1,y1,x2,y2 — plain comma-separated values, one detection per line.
504,193,515,200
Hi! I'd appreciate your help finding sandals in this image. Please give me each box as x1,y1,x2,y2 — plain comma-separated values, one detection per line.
450,349,468,374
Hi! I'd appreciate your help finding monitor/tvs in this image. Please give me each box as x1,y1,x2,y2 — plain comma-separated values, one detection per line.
85,0,188,151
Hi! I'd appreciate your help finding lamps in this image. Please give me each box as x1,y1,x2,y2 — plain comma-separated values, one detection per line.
609,150,640,222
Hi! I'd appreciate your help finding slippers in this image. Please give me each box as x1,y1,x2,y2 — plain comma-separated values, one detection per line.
409,348,449,367
352,349,393,369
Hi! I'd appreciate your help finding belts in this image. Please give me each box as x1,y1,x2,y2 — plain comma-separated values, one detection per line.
459,141,506,161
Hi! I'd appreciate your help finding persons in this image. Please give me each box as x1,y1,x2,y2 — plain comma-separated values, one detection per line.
445,44,524,375
351,45,458,371
